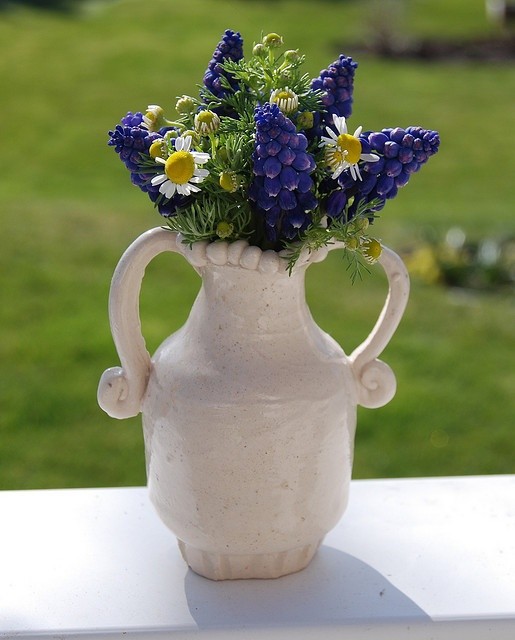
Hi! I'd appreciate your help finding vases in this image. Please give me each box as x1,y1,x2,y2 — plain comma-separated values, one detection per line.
97,228,413,581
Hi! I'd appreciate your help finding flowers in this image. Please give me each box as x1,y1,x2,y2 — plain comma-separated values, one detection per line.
108,28,439,286
